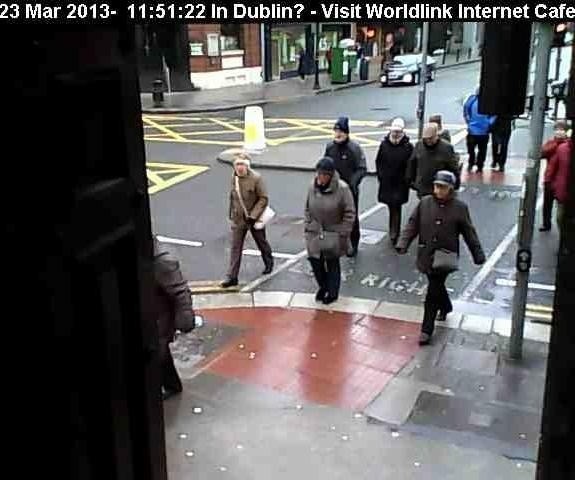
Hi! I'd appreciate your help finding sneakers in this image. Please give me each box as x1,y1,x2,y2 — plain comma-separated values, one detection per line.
261,259,275,275
346,247,358,258
538,223,553,231
418,329,433,345
435,310,448,321
218,277,239,288
315,288,339,304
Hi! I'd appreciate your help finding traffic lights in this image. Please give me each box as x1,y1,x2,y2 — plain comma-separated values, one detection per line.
555,20,568,46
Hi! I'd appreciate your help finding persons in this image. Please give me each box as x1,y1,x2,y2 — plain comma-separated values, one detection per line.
544,130,574,253
464,90,499,173
303,156,356,304
324,116,366,258
374,115,460,244
220,153,273,288
152,228,195,396
394,170,484,345
491,115,511,173
539,123,568,231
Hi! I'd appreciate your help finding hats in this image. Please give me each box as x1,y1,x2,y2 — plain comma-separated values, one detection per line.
234,152,252,167
314,156,337,177
421,114,443,139
388,117,406,133
333,116,350,134
432,169,457,188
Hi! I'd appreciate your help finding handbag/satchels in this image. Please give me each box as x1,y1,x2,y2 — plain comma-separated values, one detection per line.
253,205,277,230
431,247,460,272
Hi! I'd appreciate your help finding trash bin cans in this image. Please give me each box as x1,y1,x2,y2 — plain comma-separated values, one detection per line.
330,48,349,84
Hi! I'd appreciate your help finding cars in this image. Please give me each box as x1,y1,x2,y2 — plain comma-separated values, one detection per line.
379,51,439,86
